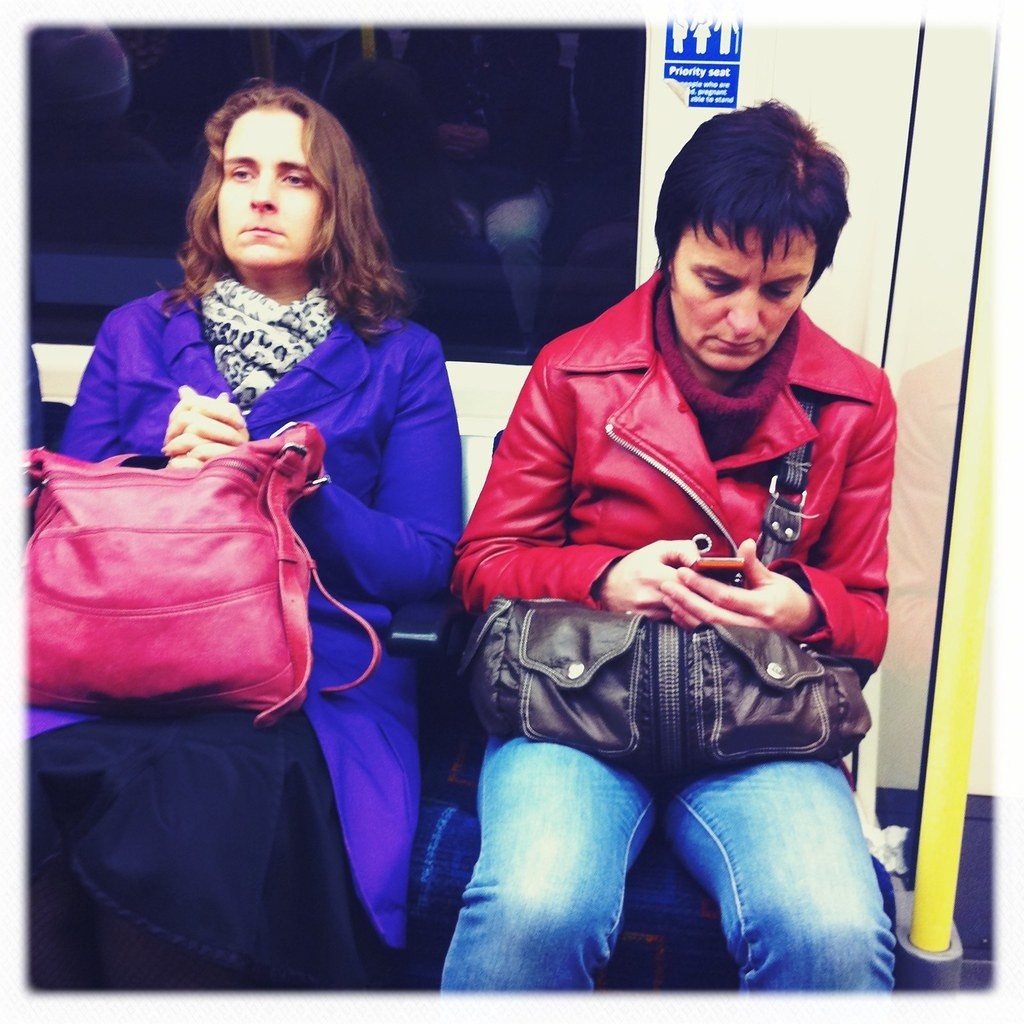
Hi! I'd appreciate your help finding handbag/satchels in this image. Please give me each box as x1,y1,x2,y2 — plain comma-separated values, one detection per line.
456,585,872,790
26,419,382,730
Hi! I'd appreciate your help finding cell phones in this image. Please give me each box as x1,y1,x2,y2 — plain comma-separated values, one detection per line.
690,557,744,582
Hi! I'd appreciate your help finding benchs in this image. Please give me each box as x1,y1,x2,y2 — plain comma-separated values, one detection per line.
39,401,908,937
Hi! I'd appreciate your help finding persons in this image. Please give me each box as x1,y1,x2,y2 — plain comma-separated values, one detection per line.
32,85,464,988
32,31,644,365
440,103,898,992
